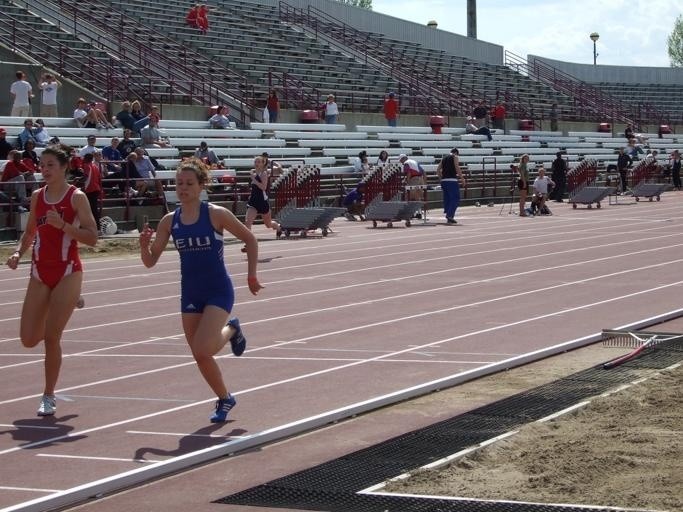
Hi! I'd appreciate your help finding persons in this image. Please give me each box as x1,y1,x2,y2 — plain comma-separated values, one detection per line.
473,101,487,129
489,100,505,129
342,148,462,226
185,5,207,31
138,156,264,422
318,94,340,124
428,108,444,135
383,93,401,127
513,141,682,218
549,104,558,131
199,4,210,32
1,72,284,255
624,123,649,146
5,144,97,415
465,116,493,142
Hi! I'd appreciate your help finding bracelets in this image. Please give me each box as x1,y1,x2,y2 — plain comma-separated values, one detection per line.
248,275,256,284
14,250,21,258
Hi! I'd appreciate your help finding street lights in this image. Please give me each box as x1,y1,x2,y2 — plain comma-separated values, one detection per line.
426,20,438,29
589,32,599,66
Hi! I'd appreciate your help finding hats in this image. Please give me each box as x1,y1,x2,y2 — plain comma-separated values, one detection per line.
88,134,95,139
399,154,406,161
389,92,394,96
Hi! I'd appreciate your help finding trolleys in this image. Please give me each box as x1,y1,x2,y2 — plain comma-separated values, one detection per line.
567,158,618,211
630,157,672,202
359,161,427,230
267,159,347,240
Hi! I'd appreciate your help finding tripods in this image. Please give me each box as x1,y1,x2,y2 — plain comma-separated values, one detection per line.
498,175,519,216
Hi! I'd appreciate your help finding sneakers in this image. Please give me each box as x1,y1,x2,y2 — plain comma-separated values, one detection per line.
210,395,236,422
277,224,281,237
227,318,247,357
123,188,139,197
38,396,57,415
97,122,115,129
242,244,247,252
447,217,456,223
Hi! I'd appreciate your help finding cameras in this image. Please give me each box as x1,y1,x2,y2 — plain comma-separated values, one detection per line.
46,74,52,79
